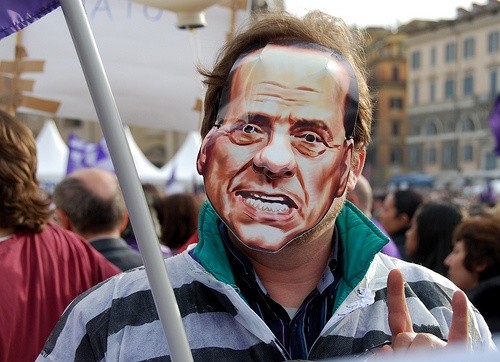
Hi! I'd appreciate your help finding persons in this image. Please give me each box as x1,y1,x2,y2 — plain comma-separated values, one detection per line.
51,168,142,273
0,110,123,362
32,9,500,362
127,181,209,251
347,169,500,339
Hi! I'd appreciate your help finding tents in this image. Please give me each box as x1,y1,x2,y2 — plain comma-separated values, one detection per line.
15,112,204,197
